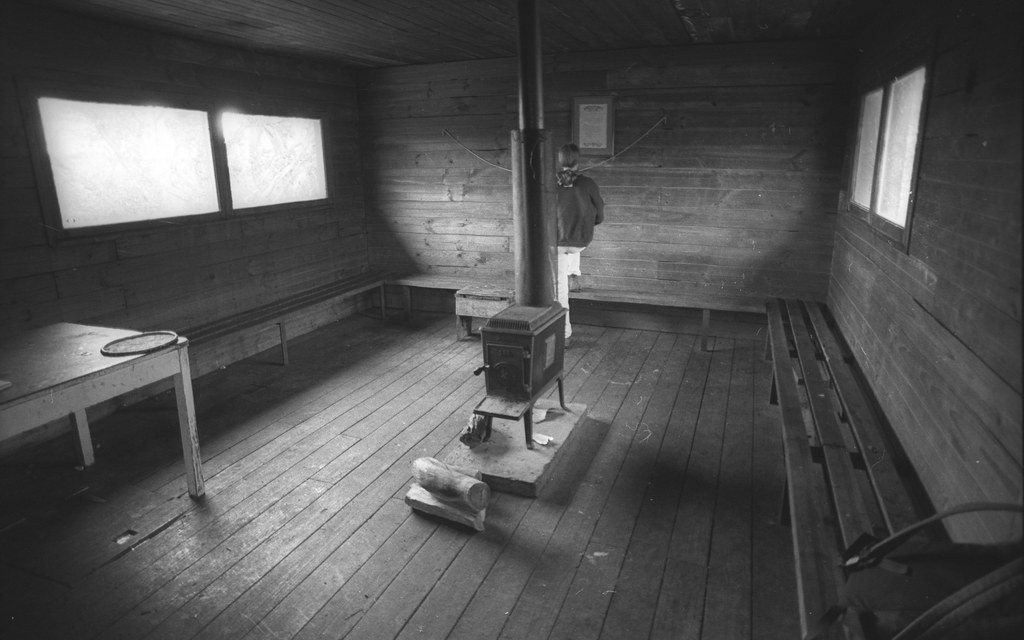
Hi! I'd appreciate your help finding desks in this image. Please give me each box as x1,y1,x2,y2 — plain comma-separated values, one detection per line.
0,322,205,499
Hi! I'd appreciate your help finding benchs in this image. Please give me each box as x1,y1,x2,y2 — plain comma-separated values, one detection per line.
391,273,766,352
176,273,387,368
766,296,931,640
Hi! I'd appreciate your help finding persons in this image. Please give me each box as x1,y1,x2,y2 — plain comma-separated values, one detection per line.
554,142,604,348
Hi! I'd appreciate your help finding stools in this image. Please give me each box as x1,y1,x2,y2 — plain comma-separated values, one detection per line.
455,283,516,341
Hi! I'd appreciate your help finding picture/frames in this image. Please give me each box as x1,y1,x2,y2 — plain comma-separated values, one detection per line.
572,95,616,158
